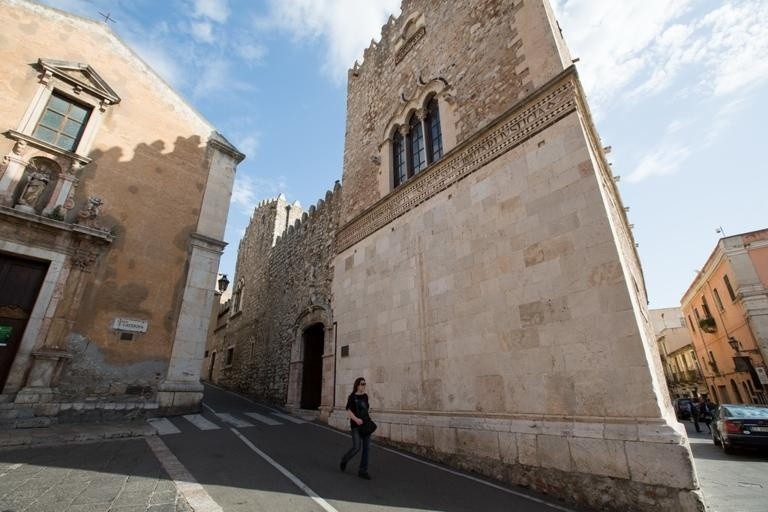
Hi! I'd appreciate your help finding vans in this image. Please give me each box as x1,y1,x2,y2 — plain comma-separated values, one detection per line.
673,398,693,420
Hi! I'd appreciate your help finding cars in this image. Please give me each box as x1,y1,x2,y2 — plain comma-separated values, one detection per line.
710,403,767,455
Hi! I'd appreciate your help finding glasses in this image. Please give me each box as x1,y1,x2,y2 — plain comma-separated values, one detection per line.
358,382,366,386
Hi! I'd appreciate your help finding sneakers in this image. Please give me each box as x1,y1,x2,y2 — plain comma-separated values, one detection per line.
361,474,371,480
340,460,346,471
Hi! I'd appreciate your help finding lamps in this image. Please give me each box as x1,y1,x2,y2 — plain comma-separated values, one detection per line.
727,336,740,352
214,273,229,296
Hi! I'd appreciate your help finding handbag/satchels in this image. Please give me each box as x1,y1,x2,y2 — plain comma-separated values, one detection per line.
359,419,377,439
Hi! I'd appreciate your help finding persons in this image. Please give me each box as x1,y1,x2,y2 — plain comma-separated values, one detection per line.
18,164,50,208
687,397,702,432
699,397,714,434
340,377,371,480
